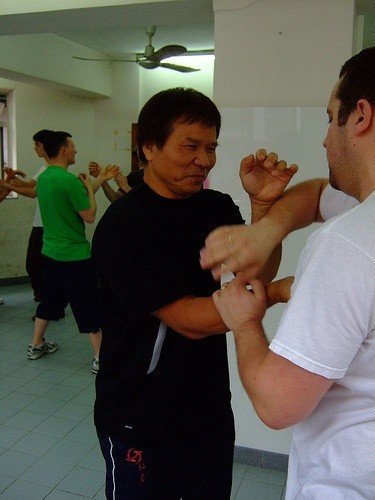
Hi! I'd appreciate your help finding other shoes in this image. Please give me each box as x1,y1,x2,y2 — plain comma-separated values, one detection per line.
29,310,66,324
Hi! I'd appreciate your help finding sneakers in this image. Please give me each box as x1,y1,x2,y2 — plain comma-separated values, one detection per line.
26,336,59,360
90,357,100,374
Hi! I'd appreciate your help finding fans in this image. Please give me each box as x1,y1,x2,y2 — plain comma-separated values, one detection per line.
72,25,214,74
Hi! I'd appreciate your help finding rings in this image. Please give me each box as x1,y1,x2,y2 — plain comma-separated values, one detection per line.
220,286,228,290
224,231,237,244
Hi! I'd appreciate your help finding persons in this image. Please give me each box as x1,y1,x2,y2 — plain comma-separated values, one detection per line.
200,48,375,500
26,131,120,373
0,130,65,321
0,165,26,306
89,150,144,203
92,87,298,500
